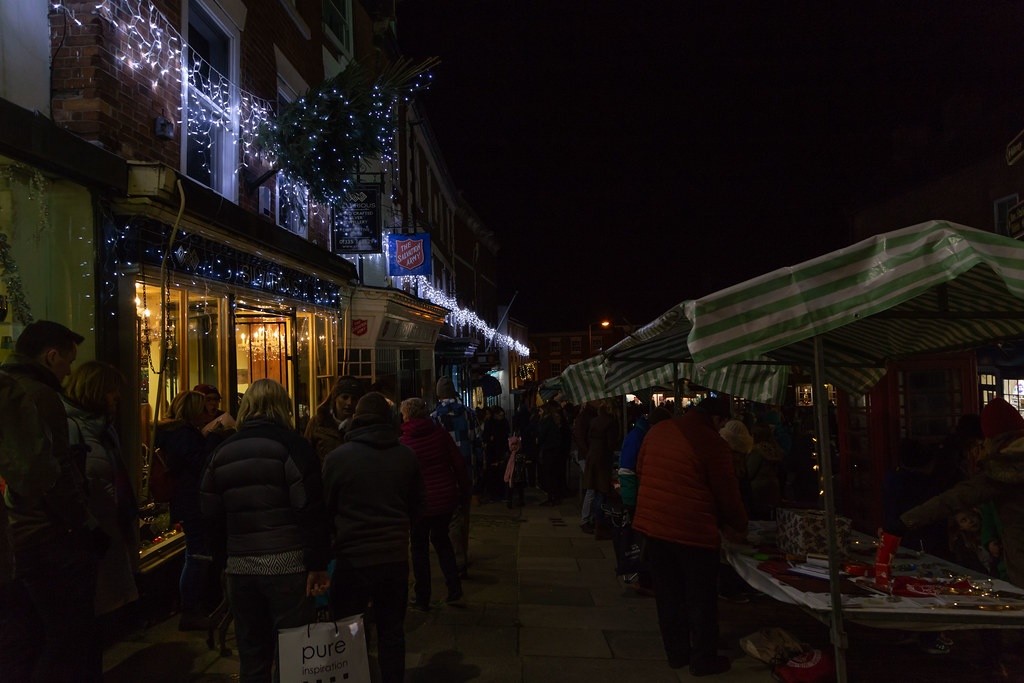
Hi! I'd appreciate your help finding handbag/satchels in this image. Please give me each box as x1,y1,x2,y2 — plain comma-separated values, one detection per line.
740,627,836,683
276,590,371,683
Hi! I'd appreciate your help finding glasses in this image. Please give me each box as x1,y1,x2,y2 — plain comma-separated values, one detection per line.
203,396,220,402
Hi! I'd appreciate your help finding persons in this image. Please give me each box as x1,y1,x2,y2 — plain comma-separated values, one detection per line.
155,376,486,683
881,398,1024,683
474,396,624,540
616,395,792,676
0,321,142,683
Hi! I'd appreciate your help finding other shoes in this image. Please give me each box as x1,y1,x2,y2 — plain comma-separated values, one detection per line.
689,656,731,676
410,597,430,610
920,637,954,655
178,614,215,631
447,597,466,607
896,632,920,645
669,653,689,668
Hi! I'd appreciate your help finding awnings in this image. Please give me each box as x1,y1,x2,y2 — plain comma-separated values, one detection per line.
604,219,1024,683
537,354,793,418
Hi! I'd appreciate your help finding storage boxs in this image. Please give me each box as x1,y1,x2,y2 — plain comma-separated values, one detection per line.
776,508,851,556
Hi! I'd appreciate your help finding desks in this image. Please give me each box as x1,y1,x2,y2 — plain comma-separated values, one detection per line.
721,521,1024,683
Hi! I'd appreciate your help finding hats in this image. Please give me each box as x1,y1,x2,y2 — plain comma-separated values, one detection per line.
980,397,1024,437
194,384,221,397
336,379,364,398
719,420,753,454
696,397,732,419
648,407,671,425
356,392,392,420
436,375,456,398
